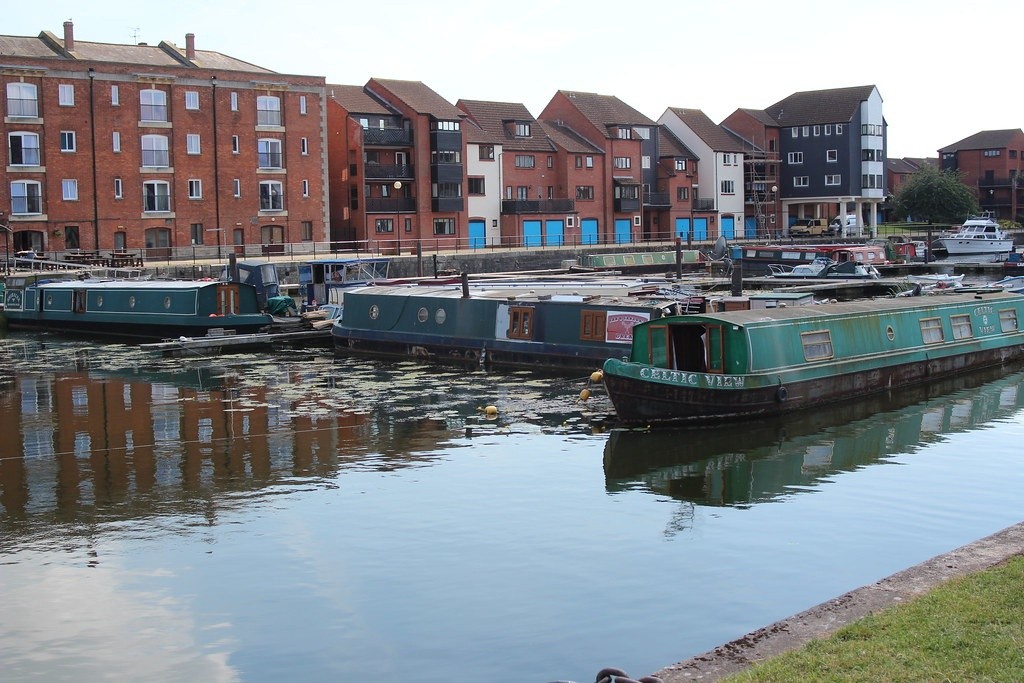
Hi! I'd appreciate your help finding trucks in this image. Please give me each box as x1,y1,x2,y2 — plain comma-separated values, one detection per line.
790,218,829,237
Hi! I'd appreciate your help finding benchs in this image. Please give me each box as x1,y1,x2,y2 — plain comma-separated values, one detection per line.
60,258,144,263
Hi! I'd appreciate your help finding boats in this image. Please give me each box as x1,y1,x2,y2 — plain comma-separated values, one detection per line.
907,274,965,288
603,290,1023,425
767,257,881,280
938,211,1014,255
604,358,1023,511
0,273,276,342
329,271,681,375
726,235,928,276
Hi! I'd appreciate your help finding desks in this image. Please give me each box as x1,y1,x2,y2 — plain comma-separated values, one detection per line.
110,252,137,268
33,256,51,269
64,255,94,270
71,252,95,255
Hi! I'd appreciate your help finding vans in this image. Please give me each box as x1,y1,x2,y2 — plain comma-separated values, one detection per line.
829,215,865,236
